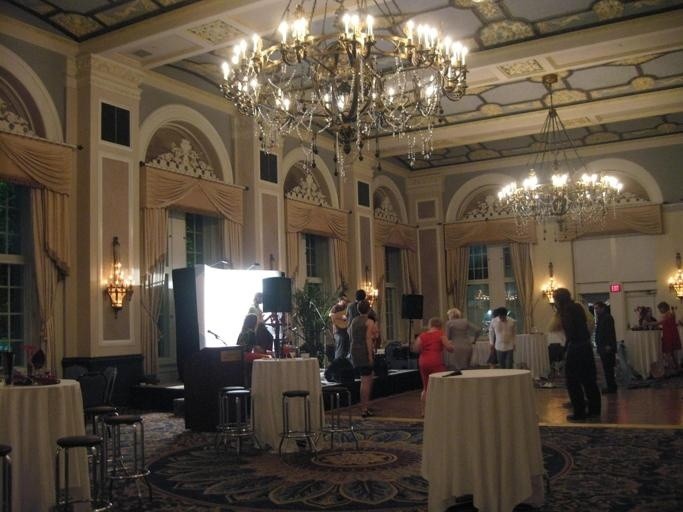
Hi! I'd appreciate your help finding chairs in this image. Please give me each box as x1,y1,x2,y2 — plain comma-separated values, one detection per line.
251,357,325,450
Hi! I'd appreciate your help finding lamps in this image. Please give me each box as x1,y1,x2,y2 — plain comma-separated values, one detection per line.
669,254,683,299
497,81,624,224
364,265,379,311
543,263,560,308
218,0,470,184
107,240,128,312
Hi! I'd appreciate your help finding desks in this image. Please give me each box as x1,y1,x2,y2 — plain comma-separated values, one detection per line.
418,368,547,511
0,378,91,512
624,328,662,379
515,333,552,378
471,338,492,368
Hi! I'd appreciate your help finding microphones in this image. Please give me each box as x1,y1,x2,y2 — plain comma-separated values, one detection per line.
308,299,312,309
207,328,228,346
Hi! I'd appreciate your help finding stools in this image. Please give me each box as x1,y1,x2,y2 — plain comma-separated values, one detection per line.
55,436,109,511
279,390,318,464
83,403,128,477
218,390,259,456
221,383,252,449
105,414,157,509
0,443,14,512
317,385,360,454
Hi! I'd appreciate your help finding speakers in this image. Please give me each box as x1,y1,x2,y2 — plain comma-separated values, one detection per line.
262,278,292,313
400,294,424,319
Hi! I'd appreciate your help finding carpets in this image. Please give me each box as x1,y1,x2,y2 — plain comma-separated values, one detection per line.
90,412,683,512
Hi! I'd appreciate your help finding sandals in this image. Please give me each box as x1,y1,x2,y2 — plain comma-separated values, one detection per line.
359,408,376,418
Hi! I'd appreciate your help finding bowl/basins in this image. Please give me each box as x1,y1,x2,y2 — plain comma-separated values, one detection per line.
301,352,310,358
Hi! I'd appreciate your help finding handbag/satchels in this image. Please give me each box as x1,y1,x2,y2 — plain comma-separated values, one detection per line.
325,357,354,383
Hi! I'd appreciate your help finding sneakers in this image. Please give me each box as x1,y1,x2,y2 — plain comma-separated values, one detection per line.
562,400,573,410
566,414,600,423
602,386,618,393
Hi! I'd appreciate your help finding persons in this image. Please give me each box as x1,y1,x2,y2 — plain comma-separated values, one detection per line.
330,289,380,417
489,307,517,368
545,288,617,421
238,292,273,353
640,301,682,377
444,308,482,369
414,317,454,416
